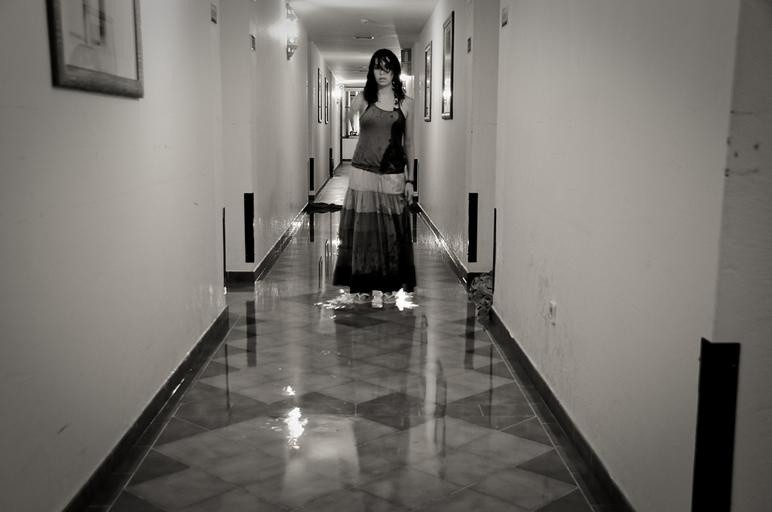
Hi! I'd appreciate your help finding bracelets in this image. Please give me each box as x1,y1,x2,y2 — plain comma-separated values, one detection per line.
406,180,415,185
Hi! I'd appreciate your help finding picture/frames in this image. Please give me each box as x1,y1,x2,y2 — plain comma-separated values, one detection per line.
423,15,455,124
47,0,144,100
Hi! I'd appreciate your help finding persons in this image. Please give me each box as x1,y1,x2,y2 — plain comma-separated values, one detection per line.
333,49,415,304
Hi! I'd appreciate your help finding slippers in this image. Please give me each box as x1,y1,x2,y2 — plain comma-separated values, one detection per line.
352,293,397,304
